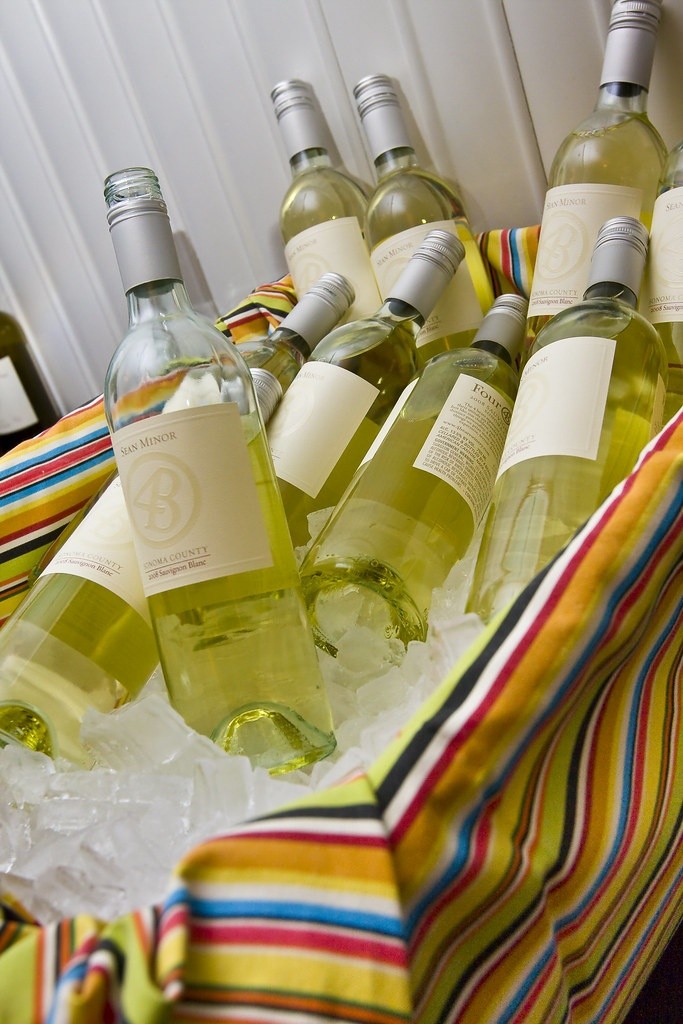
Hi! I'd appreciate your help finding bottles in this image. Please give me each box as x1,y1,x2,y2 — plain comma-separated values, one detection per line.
297,292,531,663
0,272,355,759
642,136,683,430
269,77,382,327
527,0,669,344
103,167,335,772
466,216,666,631
265,230,466,548
352,73,492,366
